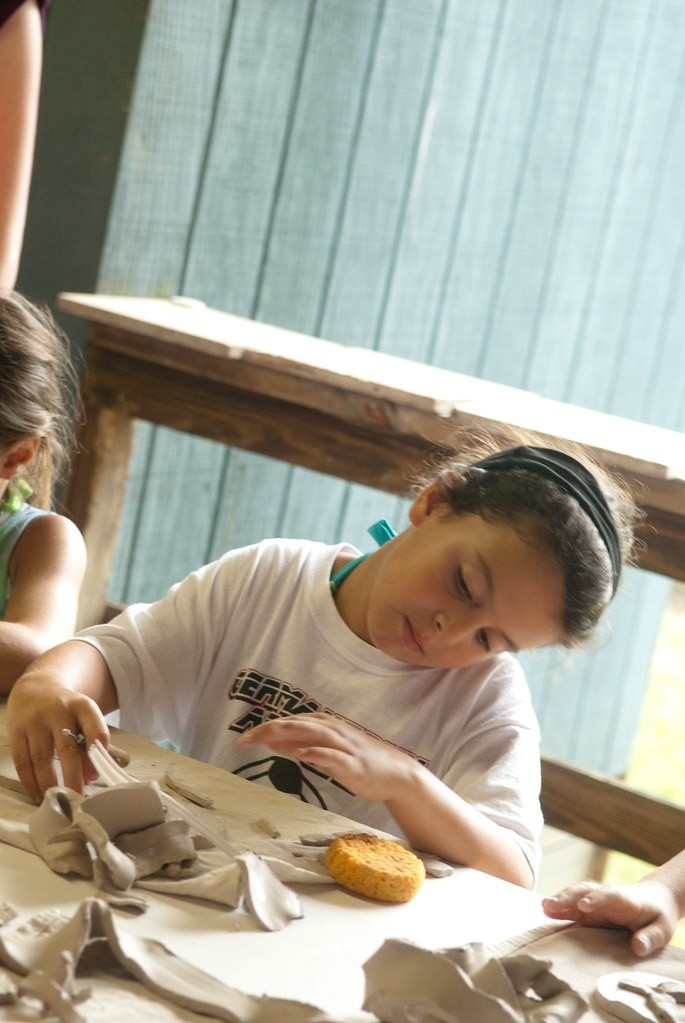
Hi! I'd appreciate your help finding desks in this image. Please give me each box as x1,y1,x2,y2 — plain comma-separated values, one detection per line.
1,692,685,1023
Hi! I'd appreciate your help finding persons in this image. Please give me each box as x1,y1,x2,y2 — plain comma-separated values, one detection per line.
0,295,85,699
541,850,685,958
4,442,631,893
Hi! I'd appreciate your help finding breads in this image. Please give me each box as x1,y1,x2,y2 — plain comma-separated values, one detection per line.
326,831,427,905
